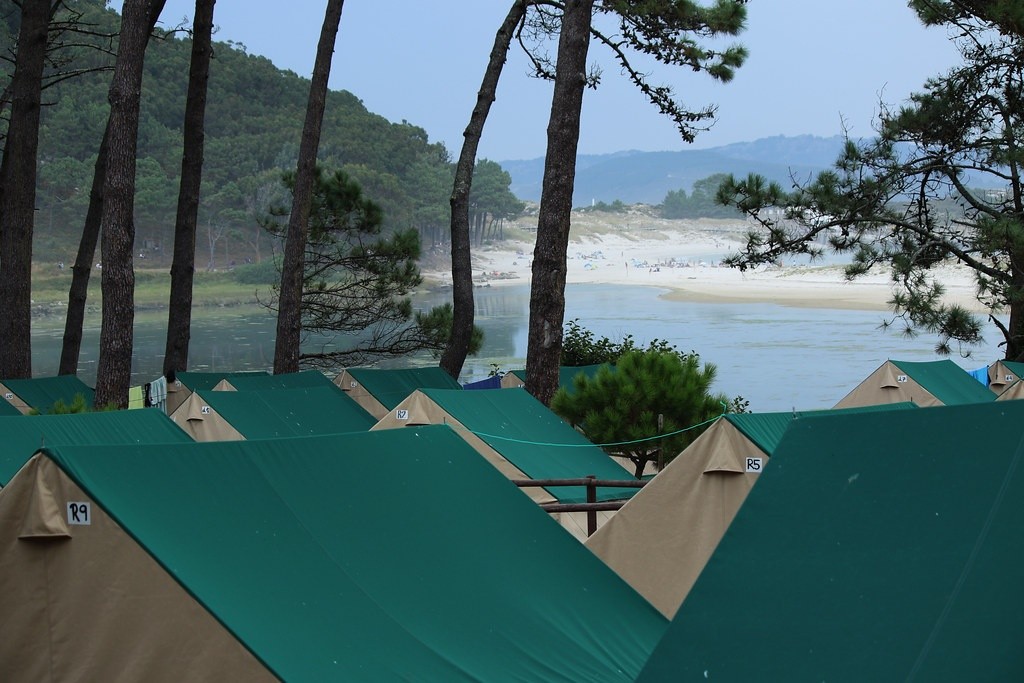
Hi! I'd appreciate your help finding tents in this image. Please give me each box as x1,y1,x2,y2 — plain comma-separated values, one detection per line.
0,360,1024,683
